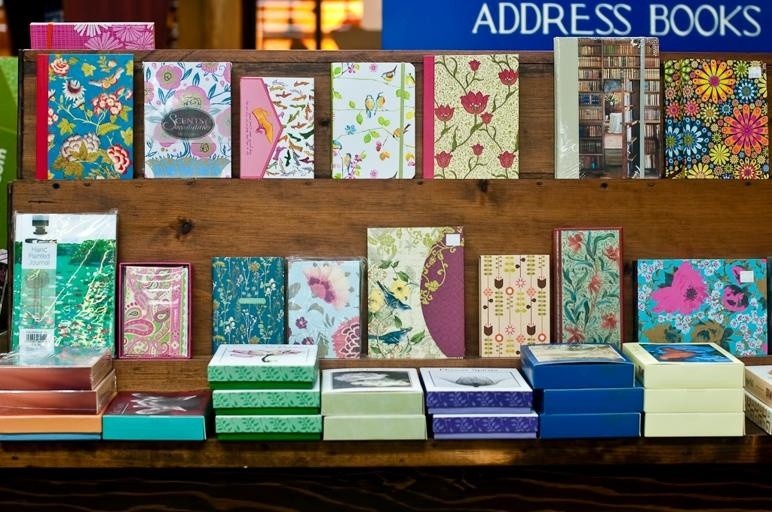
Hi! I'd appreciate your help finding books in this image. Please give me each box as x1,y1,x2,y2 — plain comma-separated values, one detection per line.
579,38,661,177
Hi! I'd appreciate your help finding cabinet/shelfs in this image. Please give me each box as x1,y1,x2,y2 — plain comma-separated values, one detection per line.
1,50,771,476
